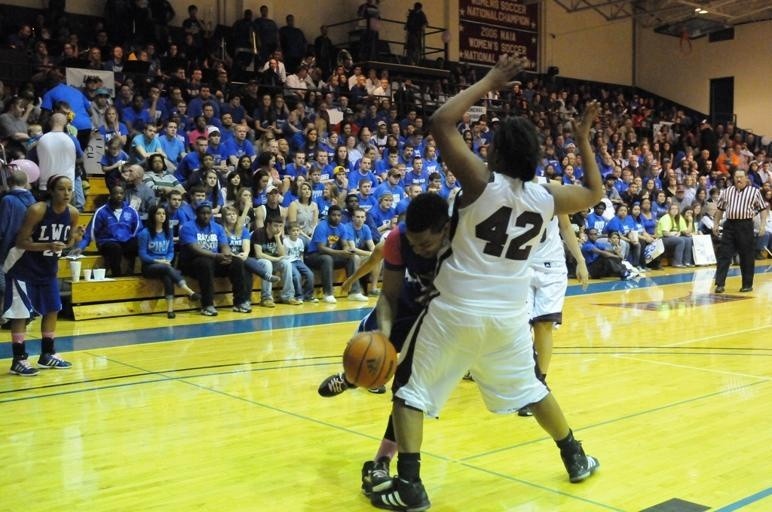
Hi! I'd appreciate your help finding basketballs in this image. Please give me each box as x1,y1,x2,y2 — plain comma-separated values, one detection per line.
343,331,397,388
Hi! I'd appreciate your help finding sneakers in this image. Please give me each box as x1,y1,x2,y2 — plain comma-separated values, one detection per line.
188,293,201,301
715,286,724,293
37,351,71,368
261,293,337,307
318,373,358,397
367,385,385,394
168,310,175,318
234,303,252,313
740,288,752,292
265,272,281,283
362,456,430,512
518,407,533,416
10,360,40,376
673,263,694,268
346,293,370,302
200,306,217,316
565,453,599,482
623,266,663,280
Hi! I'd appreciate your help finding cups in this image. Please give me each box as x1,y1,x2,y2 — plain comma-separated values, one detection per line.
69,260,82,283
630,231,639,243
82,268,92,281
93,268,107,281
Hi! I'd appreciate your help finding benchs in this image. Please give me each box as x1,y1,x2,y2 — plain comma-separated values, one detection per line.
55,175,384,321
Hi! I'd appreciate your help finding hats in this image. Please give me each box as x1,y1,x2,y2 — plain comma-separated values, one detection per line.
266,186,279,194
95,88,110,98
333,166,346,175
208,126,220,136
